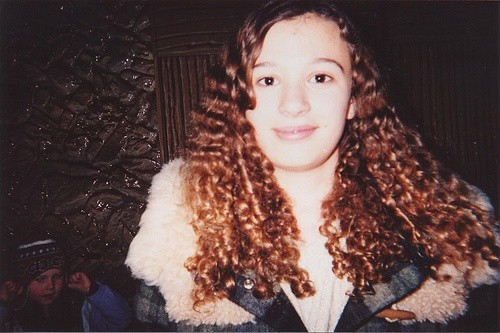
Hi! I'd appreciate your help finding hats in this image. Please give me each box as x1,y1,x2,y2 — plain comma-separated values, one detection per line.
15,233,68,311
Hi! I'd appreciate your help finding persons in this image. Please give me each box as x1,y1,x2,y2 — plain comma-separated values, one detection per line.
0,236,132,332
123,0,500,332
